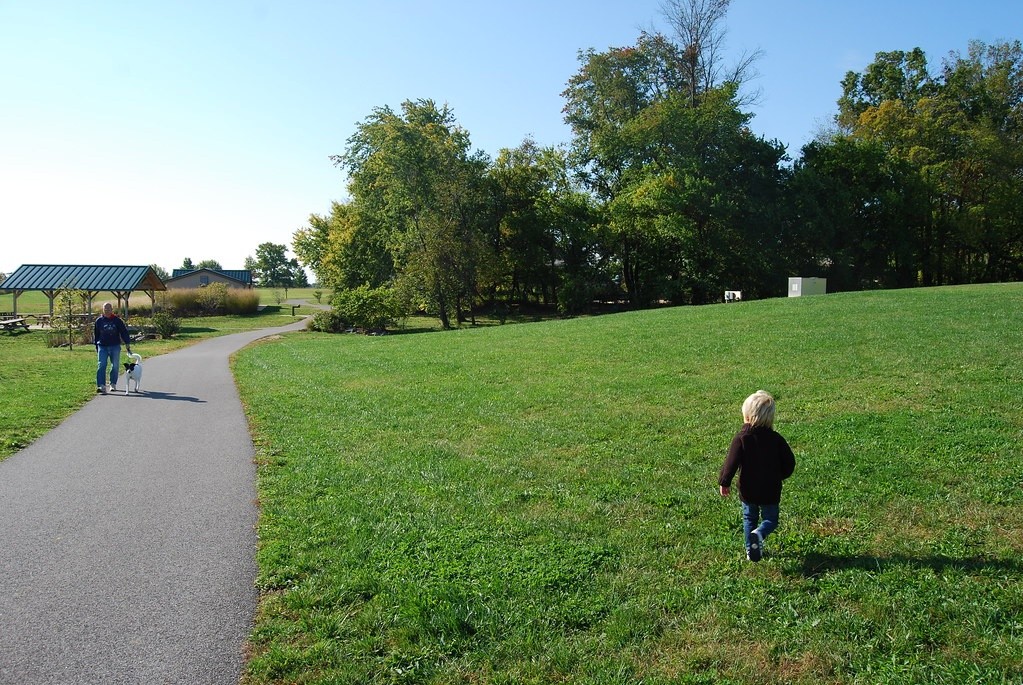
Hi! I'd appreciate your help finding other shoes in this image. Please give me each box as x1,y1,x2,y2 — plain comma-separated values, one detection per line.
110,383,116,391
97,386,107,394
747,530,766,561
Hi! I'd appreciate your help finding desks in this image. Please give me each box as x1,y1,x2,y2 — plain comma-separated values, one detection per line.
0,315,18,321
0,318,31,336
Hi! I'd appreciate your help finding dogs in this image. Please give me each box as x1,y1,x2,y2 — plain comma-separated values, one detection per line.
123,353,143,396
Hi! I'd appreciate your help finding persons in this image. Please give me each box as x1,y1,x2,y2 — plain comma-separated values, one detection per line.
717,389,797,562
93,302,132,396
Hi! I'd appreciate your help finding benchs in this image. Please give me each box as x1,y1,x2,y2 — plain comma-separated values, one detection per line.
8,325,30,331
